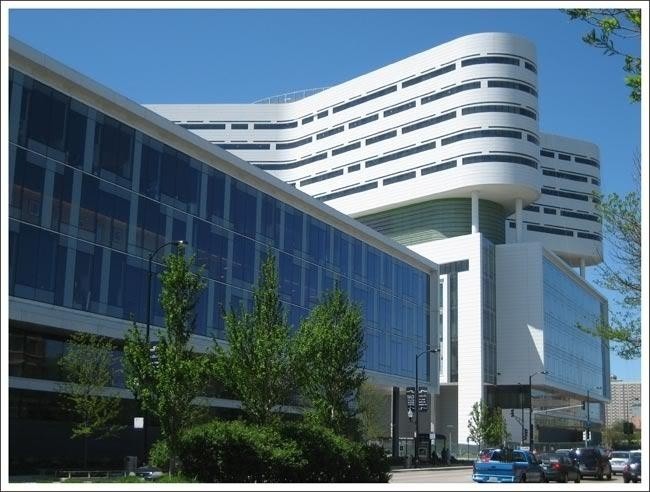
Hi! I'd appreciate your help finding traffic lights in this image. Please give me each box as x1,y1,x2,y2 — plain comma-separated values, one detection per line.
525,429,528,440
511,409,514,416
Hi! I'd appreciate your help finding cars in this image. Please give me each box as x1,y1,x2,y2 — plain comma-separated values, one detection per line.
472,447,641,483
128,465,163,481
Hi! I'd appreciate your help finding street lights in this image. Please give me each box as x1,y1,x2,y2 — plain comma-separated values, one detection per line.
467,436,473,460
587,384,603,447
143,239,189,463
528,371,548,452
446,424,455,452
626,396,639,448
408,348,440,461
